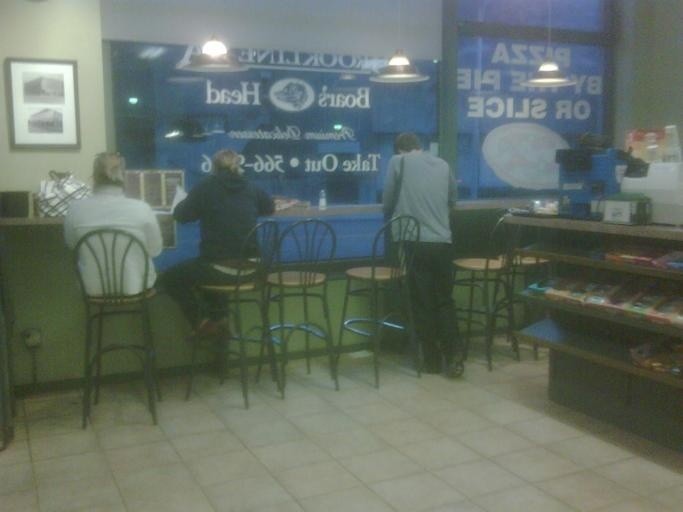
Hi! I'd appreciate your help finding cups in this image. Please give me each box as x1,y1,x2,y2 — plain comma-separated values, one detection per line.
641,132,661,165
662,124,680,168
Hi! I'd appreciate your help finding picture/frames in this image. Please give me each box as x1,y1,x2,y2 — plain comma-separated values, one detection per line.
6,56,81,151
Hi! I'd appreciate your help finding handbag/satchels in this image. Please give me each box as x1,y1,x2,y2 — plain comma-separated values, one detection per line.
33,169,91,217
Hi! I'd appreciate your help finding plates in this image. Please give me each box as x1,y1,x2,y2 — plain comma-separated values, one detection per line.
480,120,575,191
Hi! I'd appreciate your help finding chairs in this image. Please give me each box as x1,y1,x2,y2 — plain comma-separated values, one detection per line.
182,217,285,409
254,219,341,391
447,212,550,373
72,224,162,428
331,213,422,390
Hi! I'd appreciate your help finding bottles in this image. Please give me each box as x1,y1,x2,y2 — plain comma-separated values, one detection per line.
318,189,327,210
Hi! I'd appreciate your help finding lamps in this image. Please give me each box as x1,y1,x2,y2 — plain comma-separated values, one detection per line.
522,0,574,89
182,1,243,73
368,1,430,84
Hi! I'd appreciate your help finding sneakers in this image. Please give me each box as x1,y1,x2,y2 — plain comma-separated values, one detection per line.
444,339,463,379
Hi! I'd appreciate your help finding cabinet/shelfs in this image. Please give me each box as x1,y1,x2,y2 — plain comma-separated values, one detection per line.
505,215,683,454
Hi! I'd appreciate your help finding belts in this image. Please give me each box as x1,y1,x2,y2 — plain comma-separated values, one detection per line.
210,264,255,276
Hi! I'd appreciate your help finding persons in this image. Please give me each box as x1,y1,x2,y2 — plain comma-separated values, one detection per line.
382,133,469,379
61,150,162,298
159,148,276,343
